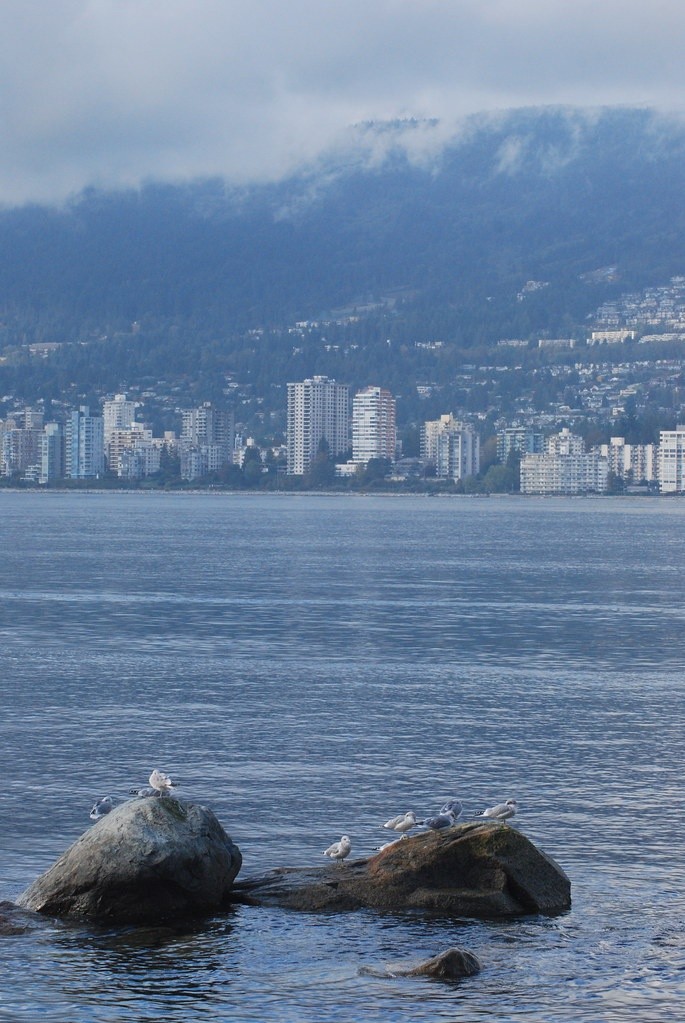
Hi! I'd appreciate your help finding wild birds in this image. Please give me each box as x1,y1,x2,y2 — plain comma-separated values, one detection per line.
383,812,415,836
474,798,517,825
322,835,352,864
439,799,464,828
414,810,458,836
149,769,172,798
